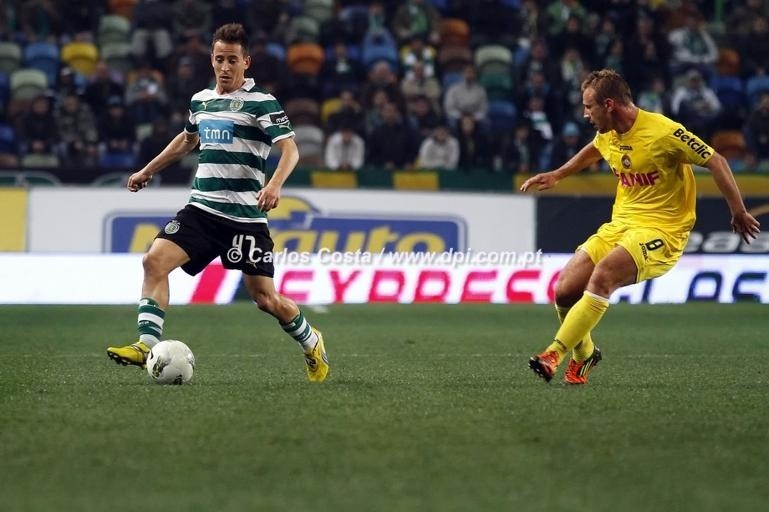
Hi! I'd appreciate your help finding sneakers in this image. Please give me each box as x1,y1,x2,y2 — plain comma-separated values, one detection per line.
302,327,329,384
528,349,561,383
563,345,603,385
106,339,152,370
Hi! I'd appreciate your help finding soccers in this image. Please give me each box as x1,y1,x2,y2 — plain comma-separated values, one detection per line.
146,339,195,385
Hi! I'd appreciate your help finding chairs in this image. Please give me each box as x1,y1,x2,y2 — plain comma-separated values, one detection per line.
0,0,769,171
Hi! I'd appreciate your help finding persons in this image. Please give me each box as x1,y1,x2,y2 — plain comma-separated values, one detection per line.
519,66,760,386
1,0,769,176
103,23,331,384
